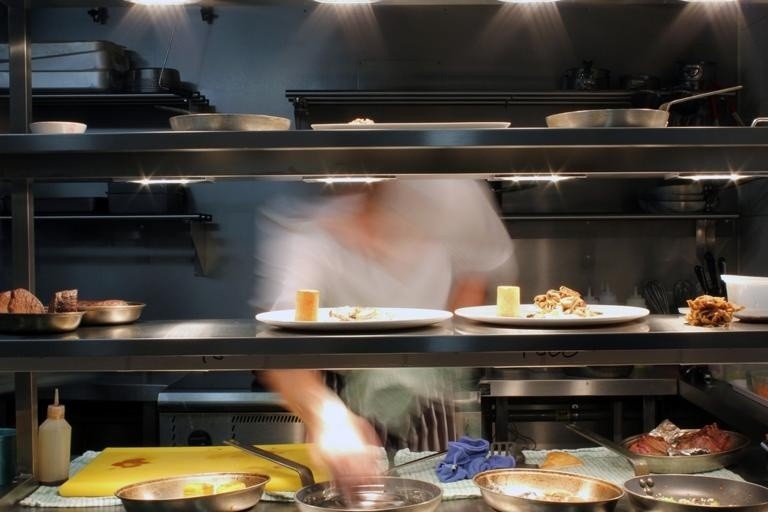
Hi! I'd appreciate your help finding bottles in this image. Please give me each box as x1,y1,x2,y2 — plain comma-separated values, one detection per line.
582,285,647,308
37,388,72,486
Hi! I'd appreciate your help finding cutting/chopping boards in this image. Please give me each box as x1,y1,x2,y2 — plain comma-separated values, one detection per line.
59,443,332,497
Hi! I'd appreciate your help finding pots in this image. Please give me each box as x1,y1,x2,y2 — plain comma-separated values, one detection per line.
636,177,768,215
560,56,611,91
221,436,441,511
545,85,742,127
566,421,768,512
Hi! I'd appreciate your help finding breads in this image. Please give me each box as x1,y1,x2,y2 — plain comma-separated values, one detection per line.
0,288,81,313
81,296,139,313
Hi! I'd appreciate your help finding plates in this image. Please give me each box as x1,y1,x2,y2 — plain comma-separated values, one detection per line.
29,120,87,134
253,307,453,330
454,304,649,325
311,121,511,129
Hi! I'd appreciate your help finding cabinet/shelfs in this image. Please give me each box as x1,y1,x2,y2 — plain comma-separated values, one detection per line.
0,85,221,279
281,85,743,250
0,0,768,512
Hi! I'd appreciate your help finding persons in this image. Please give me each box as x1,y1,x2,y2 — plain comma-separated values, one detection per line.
251,176,521,512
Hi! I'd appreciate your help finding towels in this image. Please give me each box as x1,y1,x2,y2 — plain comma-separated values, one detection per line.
435,436,517,484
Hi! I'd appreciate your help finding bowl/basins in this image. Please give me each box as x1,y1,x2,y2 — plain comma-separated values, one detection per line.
77,302,145,325
620,428,751,474
719,275,768,320
672,79,706,90
114,473,270,512
170,114,291,131
1,312,84,333
473,468,625,512
751,369,768,401
